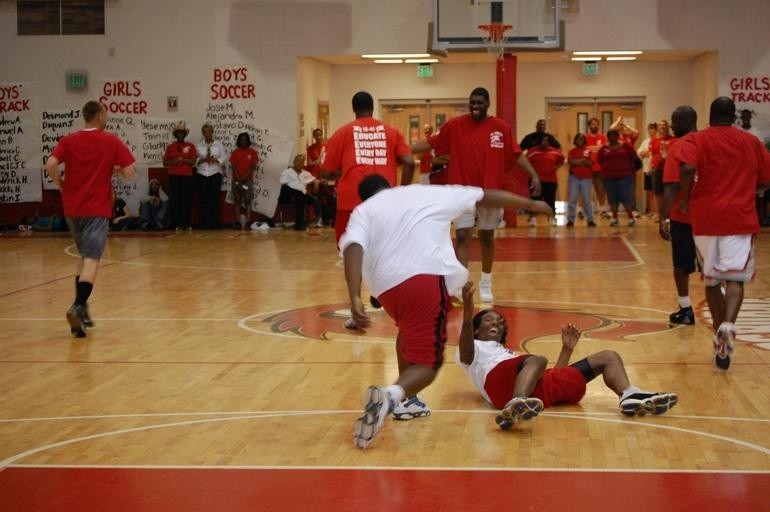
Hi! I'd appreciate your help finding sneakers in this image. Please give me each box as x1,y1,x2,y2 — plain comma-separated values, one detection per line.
353,384,388,451
496,215,638,227
479,279,494,303
65,303,93,339
618,390,678,418
392,394,433,421
669,304,695,325
711,320,736,371
495,398,544,431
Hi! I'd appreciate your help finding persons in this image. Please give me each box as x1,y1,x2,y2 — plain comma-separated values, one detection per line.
228,132,260,230
418,123,448,185
139,178,172,231
739,111,768,229
319,84,418,334
42,101,139,339
107,183,131,231
519,115,674,229
338,170,560,452
163,126,197,232
410,83,542,307
448,275,680,434
656,106,713,325
23,209,69,232
194,123,226,230
655,94,768,370
279,128,336,230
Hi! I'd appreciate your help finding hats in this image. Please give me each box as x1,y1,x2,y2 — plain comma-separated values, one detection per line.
172,123,189,135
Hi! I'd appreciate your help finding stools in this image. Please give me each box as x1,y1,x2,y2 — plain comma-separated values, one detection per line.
278,202,308,228
